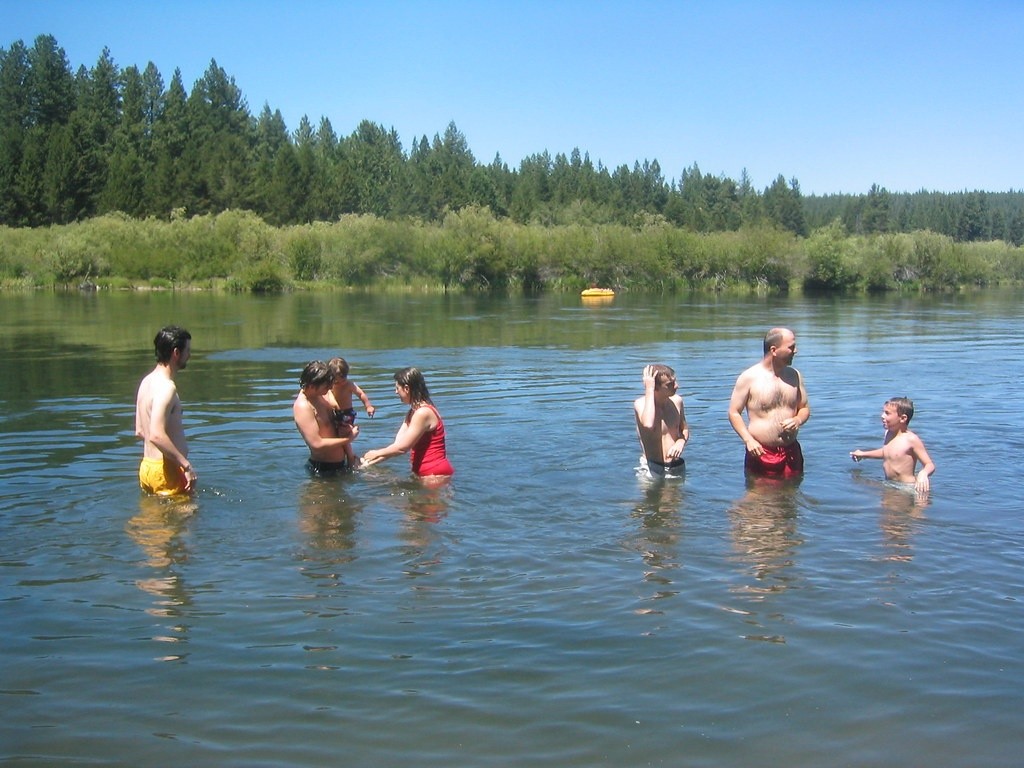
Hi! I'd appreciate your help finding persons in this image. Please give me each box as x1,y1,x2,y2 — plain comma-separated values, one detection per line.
849,396,935,494
321,358,375,467
293,360,359,480
135,326,198,497
361,367,453,482
728,328,811,481
633,365,689,480
